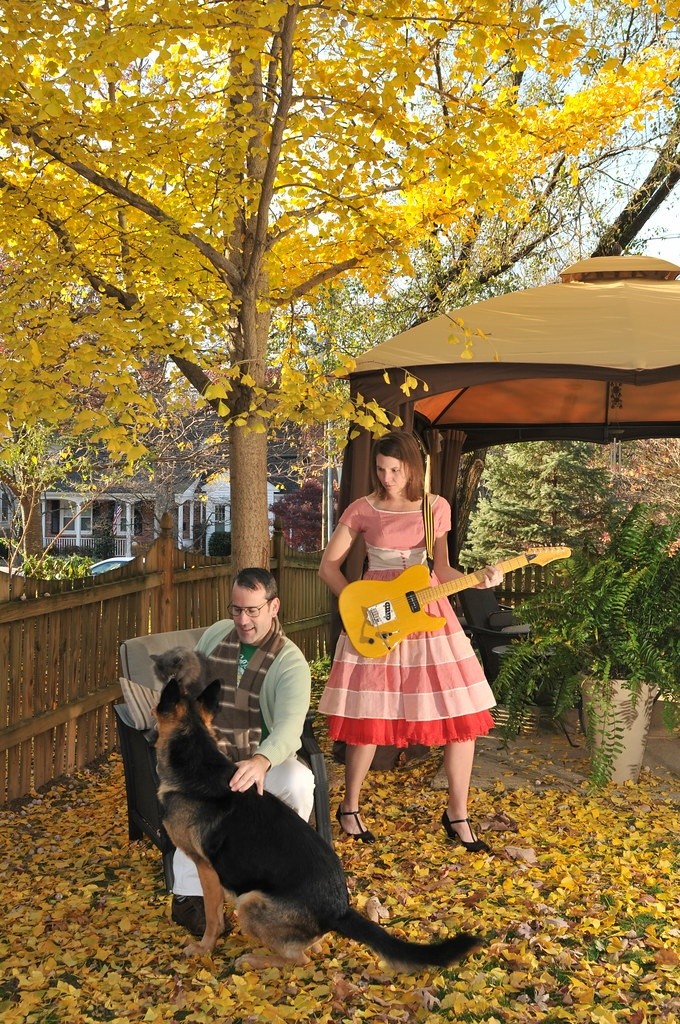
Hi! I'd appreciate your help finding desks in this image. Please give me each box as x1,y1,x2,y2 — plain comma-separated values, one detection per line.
500,624,533,643
492,645,555,712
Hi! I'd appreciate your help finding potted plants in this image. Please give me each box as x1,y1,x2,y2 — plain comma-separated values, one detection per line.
489,500,679,790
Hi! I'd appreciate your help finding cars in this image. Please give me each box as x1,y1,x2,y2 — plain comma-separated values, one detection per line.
88,557,145,576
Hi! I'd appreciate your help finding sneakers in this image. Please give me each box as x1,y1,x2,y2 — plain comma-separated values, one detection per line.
172,895,231,939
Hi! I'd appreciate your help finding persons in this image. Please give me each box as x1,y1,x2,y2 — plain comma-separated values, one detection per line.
172,568,315,936
316,431,493,853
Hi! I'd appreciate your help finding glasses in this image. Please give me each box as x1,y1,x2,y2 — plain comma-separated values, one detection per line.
227,599,269,618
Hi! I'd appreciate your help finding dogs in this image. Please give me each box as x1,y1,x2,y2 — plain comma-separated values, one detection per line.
152,677,483,973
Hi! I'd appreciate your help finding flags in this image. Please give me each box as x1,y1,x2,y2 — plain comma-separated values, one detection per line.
113,506,123,536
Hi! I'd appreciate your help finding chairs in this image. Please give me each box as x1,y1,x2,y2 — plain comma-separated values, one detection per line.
516,654,587,748
114,624,335,893
461,588,525,682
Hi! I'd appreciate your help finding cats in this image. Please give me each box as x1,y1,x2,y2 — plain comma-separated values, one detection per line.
145,645,212,741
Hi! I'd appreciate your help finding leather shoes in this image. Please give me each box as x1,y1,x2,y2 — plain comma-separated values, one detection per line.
440,808,493,857
335,803,376,845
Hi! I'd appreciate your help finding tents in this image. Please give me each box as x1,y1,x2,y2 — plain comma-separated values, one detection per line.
331,257,680,669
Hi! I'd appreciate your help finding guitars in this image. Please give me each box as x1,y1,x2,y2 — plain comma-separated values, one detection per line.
337,544,572,659
